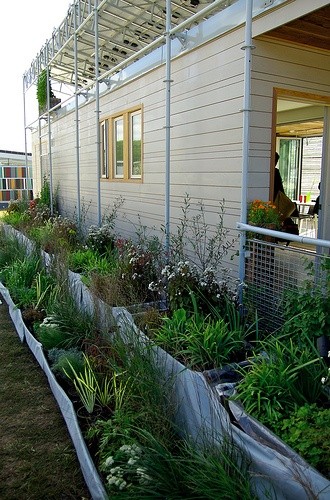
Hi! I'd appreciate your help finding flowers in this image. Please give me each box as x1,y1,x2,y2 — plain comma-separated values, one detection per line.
248,200,283,231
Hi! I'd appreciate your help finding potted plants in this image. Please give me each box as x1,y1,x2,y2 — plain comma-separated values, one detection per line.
23,304,247,499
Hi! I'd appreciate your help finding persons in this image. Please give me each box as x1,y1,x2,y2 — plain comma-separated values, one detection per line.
273,151,286,204
312,182,320,217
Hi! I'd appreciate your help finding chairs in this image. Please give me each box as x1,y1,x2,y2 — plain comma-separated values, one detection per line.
303,213,319,236
290,202,312,233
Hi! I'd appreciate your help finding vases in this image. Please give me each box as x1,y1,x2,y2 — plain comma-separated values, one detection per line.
261,227,278,246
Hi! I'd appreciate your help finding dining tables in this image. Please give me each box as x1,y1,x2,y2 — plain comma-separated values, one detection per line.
293,201,318,238
